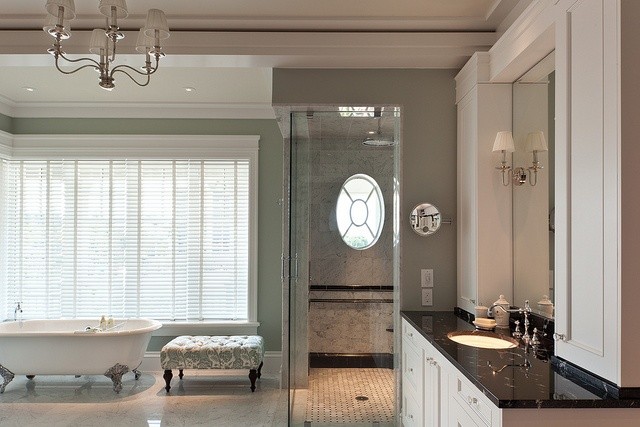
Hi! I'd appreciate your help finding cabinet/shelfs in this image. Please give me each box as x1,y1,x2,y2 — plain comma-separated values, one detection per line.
432,345,502,427
401,317,431,427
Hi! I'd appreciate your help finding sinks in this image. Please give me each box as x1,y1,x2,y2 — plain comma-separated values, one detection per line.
445,330,519,349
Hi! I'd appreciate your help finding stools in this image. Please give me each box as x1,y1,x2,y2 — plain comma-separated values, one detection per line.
160,335,265,394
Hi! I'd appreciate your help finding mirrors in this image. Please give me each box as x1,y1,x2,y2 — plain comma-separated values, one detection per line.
409,202,441,237
512,48,556,323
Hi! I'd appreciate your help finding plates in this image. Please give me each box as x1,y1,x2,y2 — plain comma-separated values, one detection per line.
472,322,498,331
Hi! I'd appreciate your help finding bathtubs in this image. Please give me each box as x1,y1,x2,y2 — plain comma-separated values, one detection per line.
0,318,162,394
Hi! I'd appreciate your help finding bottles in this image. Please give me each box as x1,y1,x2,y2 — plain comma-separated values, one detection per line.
537,295,553,319
494,295,510,329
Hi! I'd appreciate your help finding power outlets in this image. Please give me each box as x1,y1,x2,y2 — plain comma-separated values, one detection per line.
422,289,432,306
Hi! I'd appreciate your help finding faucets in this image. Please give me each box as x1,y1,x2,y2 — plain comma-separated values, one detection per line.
487,300,532,346
13,302,23,322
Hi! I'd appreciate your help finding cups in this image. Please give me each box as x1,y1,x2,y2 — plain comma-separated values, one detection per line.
474,306,488,318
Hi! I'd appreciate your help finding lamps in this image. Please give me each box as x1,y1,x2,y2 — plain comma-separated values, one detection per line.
521,132,548,186
491,131,524,187
362,116,394,148
336,172,386,250
42,0,170,91
